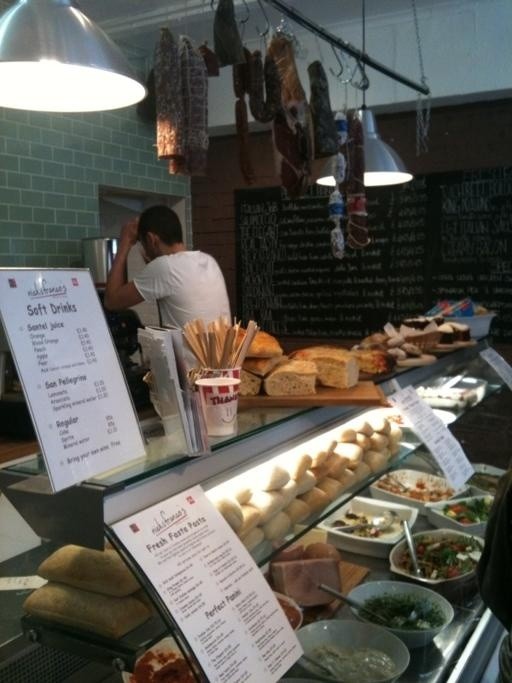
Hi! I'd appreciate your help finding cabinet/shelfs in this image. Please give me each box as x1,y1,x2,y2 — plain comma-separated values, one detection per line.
1,334,512,683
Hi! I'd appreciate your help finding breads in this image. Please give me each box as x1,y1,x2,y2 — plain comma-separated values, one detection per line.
235,314,470,397
22,410,402,642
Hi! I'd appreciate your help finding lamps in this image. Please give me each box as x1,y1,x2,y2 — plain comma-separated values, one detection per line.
313,53,415,190
1,0,150,115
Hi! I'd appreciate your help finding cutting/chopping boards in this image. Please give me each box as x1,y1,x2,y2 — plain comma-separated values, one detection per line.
235,376,388,408
262,558,370,625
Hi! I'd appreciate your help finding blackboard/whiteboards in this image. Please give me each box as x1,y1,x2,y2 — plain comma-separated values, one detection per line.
235,165,512,340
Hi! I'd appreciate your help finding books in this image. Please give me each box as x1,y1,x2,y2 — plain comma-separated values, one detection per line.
133,322,199,457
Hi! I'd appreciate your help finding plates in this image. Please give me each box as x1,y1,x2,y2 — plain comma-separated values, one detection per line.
131,636,208,682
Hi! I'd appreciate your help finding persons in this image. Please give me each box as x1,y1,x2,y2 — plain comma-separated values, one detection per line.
474,467,512,683
104,204,233,330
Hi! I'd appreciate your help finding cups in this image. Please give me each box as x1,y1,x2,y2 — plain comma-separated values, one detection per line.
192,366,242,438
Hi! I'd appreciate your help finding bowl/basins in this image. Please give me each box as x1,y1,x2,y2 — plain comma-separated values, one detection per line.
346,580,454,646
298,618,412,683
390,526,488,589
321,494,420,562
370,469,452,514
427,486,495,536
